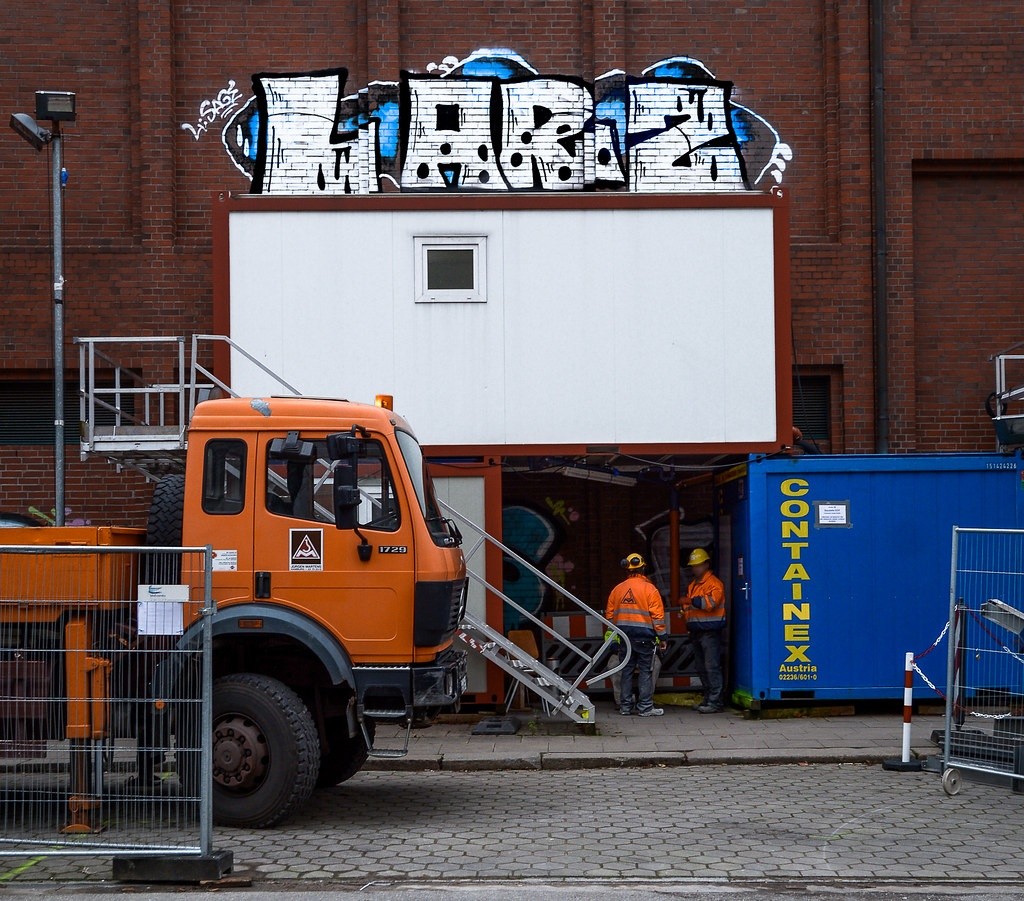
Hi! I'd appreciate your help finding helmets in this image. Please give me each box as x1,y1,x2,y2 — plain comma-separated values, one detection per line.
625,553,647,569
687,549,711,565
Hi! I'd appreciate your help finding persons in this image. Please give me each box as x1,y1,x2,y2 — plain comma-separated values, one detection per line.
604,553,667,717
677,548,728,712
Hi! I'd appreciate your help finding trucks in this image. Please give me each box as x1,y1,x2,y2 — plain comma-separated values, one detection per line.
0,397,472,830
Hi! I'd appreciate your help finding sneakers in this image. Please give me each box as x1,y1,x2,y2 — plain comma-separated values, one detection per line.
638,707,664,716
692,701,707,709
699,702,723,713
619,709,630,714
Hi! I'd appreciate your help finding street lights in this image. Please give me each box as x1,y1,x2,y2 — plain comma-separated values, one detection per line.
33,88,78,524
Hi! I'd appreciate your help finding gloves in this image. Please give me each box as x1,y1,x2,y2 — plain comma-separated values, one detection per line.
679,596,692,605
